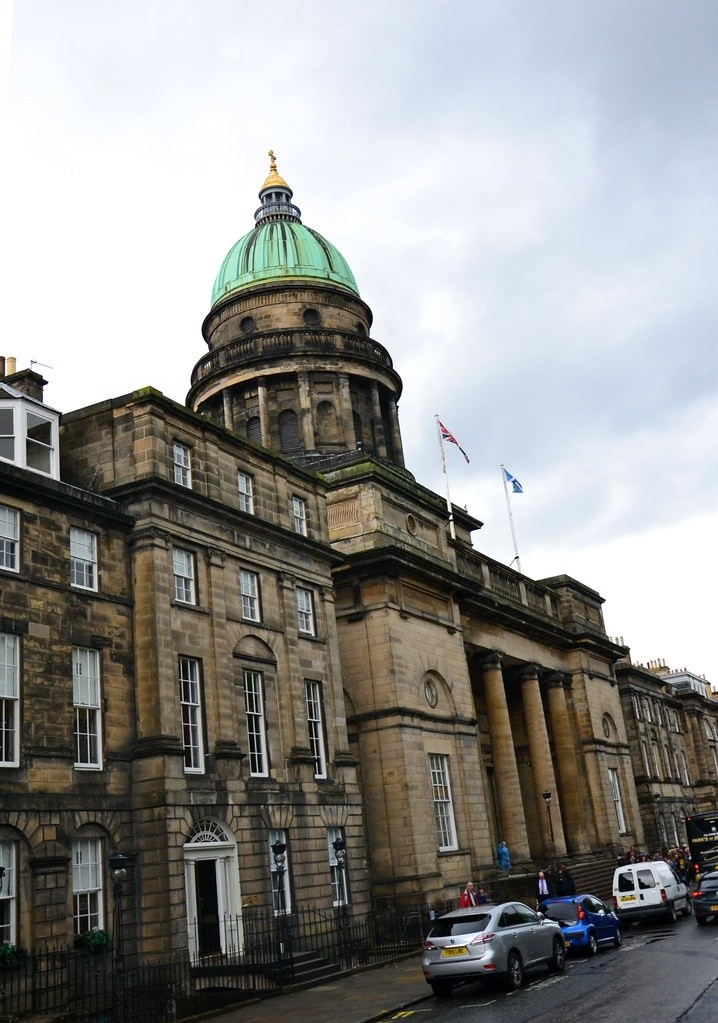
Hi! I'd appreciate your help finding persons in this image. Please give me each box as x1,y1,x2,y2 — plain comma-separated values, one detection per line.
557,862,576,896
461,880,490,909
499,842,512,872
536,870,554,912
617,844,696,887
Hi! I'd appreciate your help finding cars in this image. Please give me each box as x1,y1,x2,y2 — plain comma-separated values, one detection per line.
535,893,622,956
690,869,718,926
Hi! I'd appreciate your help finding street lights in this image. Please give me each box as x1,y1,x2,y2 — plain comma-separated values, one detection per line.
542,790,559,867
108,851,131,1023
271,839,295,984
331,837,353,970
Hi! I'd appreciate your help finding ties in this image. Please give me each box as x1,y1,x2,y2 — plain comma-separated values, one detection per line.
541,880,544,895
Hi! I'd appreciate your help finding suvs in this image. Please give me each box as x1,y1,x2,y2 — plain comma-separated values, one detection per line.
421,900,567,998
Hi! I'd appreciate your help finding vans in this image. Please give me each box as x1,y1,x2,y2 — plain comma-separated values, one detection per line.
612,860,692,930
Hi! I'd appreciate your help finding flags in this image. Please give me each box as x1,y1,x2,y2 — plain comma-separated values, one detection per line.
500,464,524,493
436,415,470,463
